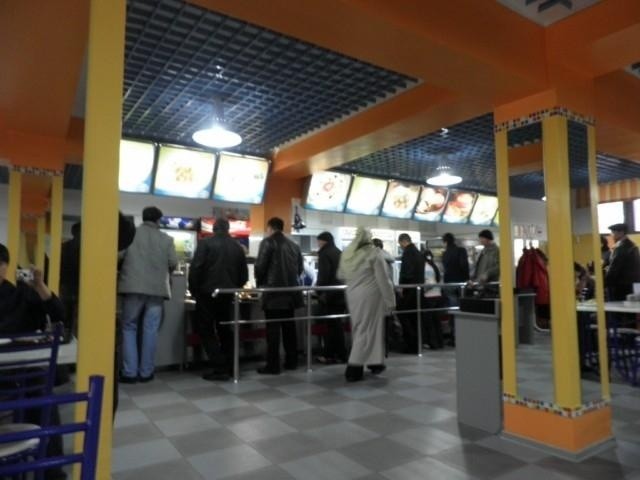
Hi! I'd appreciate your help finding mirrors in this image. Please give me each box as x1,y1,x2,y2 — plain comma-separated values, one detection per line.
504,120,557,406
566,117,607,405
17,172,54,295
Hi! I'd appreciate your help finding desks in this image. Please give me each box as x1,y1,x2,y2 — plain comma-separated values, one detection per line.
576,298,598,313
0,333,78,373
604,301,640,327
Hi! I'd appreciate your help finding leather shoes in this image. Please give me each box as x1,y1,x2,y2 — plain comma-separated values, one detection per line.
205,354,387,382
118,376,137,383
140,373,153,382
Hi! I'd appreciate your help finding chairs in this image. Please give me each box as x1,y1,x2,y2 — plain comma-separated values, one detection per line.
0,319,65,480
1,375,105,480
605,299,640,386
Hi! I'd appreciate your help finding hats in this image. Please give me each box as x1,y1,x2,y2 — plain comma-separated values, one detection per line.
479,230,493,240
608,223,628,232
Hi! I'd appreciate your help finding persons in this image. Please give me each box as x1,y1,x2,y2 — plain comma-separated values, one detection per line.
395,229,500,355
0,244,67,380
600,223,638,302
311,224,396,382
58,206,176,381
254,218,303,376
187,218,248,380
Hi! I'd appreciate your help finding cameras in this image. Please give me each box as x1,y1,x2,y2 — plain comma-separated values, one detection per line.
16,268,34,283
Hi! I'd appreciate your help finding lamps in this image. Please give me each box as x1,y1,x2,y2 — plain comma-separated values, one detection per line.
425,150,463,188
191,93,243,151
